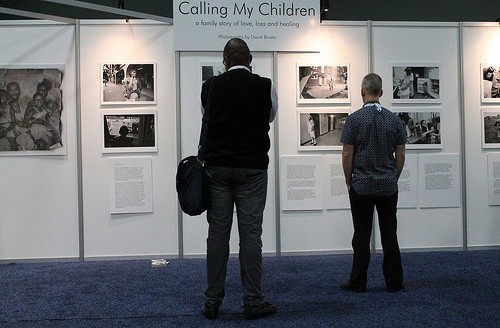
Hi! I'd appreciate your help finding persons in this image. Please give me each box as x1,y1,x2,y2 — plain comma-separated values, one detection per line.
198,39,278,320
102,68,145,146
340,73,408,292
0,79,63,152
306,67,440,144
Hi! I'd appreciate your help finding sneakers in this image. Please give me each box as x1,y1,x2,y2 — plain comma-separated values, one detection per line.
201,305,219,319
244,303,277,320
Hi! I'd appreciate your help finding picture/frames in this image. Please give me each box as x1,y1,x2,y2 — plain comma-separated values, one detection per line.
100,108,158,155
0,62,67,156
390,61,443,104
390,107,443,150
99,59,157,106
480,62,500,104
200,61,256,87
295,62,351,105
296,108,351,151
481,108,500,149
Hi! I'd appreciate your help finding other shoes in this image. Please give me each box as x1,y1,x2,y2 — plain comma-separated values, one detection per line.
340,281,354,291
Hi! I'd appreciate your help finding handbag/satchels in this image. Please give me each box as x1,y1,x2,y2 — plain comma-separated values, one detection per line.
176,155,210,216
